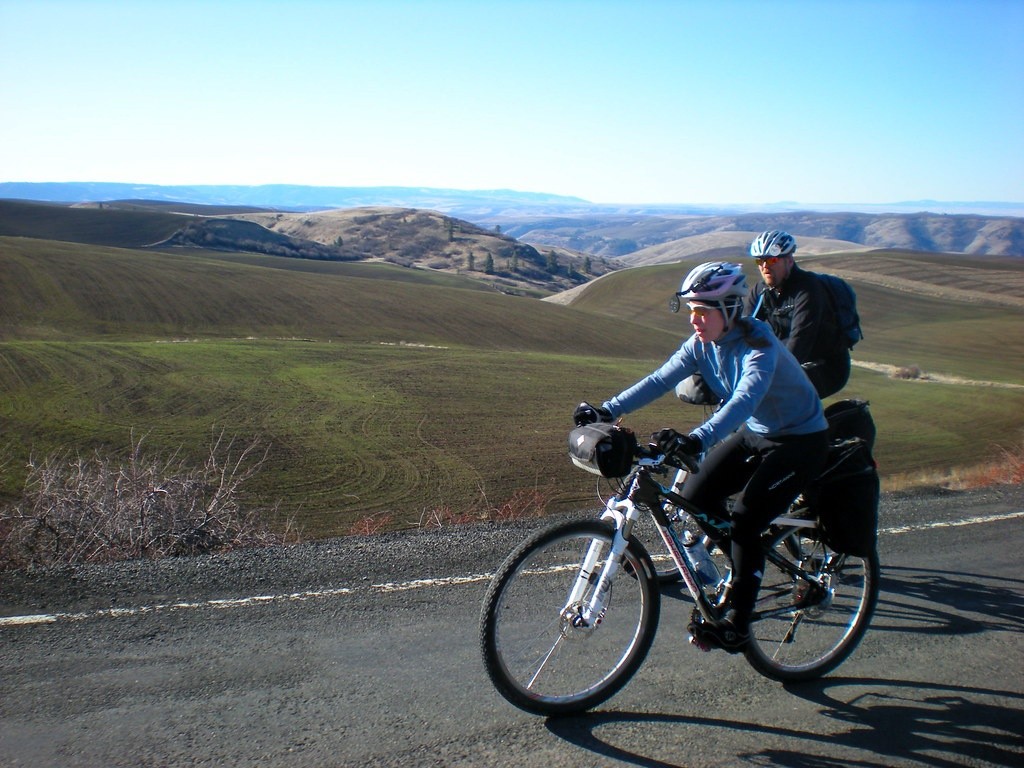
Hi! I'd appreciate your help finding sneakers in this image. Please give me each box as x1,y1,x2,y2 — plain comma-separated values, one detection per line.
687,619,755,655
714,563,735,608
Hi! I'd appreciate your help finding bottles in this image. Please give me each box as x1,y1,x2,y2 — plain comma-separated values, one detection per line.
681,530,722,587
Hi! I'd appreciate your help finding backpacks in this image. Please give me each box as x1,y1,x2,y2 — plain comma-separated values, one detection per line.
805,437,879,557
756,273,864,352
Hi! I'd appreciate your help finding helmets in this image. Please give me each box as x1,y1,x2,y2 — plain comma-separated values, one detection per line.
680,260,750,302
751,230,798,257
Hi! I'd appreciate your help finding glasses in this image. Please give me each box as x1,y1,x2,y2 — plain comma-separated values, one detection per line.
753,256,791,265
684,303,716,319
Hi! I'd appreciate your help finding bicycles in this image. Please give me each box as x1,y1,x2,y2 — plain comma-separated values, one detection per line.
478,402,882,720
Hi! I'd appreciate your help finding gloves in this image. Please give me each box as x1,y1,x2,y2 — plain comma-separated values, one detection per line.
573,400,613,427
650,427,702,458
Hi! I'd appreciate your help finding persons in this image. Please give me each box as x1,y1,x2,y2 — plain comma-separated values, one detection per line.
574,263,830,654
742,229,853,400
